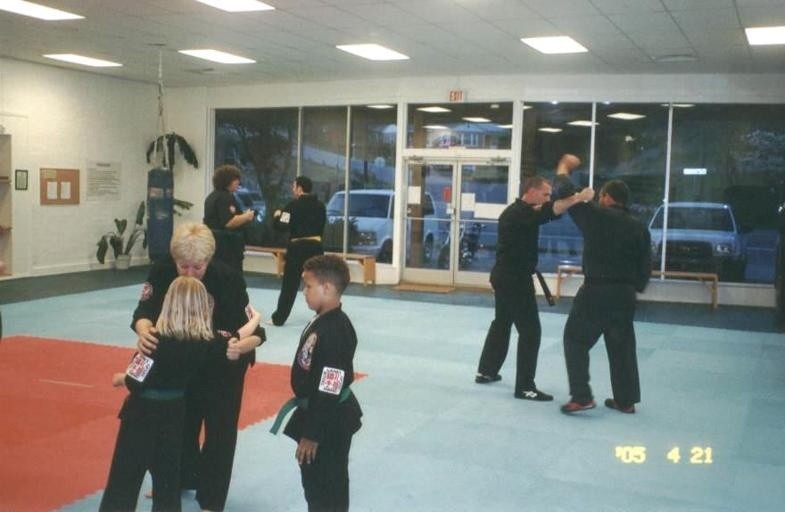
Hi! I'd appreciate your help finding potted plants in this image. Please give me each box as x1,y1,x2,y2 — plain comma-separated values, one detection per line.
96,200,148,271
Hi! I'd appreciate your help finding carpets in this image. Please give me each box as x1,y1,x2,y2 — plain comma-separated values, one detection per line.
391,279,455,293
1,335,366,512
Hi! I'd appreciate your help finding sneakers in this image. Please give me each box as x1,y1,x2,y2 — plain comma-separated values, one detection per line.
513,389,555,404
476,374,502,384
606,398,641,415
560,401,597,415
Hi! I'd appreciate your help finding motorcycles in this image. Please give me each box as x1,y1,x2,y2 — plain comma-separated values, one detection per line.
437,220,486,269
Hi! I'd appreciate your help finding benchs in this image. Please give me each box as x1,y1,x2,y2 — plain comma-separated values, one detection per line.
556,265,719,311
276,249,376,286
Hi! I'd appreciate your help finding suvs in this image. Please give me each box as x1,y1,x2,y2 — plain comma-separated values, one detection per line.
647,201,749,282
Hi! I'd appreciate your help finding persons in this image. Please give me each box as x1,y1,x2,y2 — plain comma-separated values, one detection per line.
130,223,266,512
475,176,595,401
554,154,651,414
265,176,327,326
269,254,363,512
203,164,255,273
99,276,261,512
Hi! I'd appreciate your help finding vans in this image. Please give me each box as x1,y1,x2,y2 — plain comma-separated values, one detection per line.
322,190,438,267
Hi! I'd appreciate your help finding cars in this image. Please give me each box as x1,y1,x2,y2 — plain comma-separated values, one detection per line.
235,185,267,223
